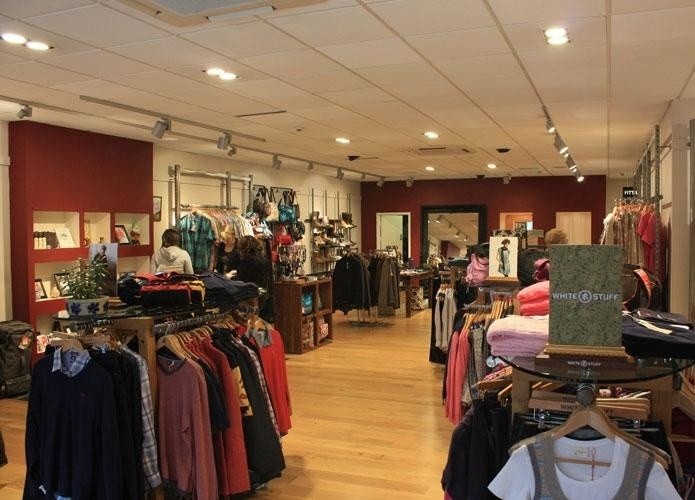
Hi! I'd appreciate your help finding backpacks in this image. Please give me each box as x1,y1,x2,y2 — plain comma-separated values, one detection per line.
0,319,33,398
120,269,259,306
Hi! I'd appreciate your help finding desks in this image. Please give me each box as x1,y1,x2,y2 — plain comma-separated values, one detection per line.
400,271,433,318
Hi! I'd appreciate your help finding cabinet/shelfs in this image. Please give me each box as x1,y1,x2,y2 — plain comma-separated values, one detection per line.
8,120,154,368
272,277,334,354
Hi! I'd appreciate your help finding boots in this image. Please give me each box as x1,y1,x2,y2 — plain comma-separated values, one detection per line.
312,210,357,263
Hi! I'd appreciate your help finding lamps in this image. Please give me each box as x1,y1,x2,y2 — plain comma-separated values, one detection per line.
270,153,385,188
149,116,232,151
546,118,584,183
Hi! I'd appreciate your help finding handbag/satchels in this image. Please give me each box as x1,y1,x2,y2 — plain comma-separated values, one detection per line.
254,187,306,283
465,242,489,287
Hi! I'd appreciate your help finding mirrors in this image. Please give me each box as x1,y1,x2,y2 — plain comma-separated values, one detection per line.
420,204,488,264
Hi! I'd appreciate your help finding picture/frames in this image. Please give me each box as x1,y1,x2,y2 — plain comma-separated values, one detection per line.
35,272,73,299
152,196,162,222
114,225,131,245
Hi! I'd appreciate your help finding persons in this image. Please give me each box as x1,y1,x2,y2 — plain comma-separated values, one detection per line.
544,228,568,252
150,229,195,275
496,239,511,277
215,225,273,310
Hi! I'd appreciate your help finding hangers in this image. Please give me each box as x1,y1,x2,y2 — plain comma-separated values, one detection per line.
506,375,695,470
461,291,512,333
59,321,83,355
156,321,213,361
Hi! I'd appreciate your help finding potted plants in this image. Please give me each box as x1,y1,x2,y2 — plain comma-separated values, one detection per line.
63,253,110,315
